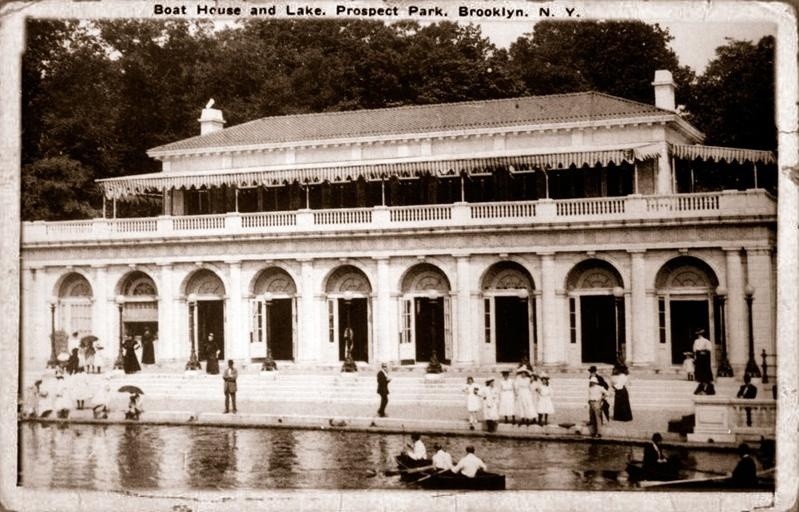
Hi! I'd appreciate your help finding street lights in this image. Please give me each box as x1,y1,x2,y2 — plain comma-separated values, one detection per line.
262,291,276,370
341,290,358,372
612,286,624,360
115,295,126,368
186,293,202,370
425,289,443,373
518,288,534,373
46,296,60,366
715,283,761,377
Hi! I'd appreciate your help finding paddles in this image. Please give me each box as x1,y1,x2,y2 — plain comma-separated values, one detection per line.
384,466,432,477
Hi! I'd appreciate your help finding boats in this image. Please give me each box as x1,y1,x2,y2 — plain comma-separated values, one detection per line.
395,455,505,490
624,461,679,482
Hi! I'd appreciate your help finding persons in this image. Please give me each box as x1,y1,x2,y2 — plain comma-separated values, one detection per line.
643,432,669,480
737,375,757,426
731,443,757,489
21,324,155,421
462,362,555,431
694,378,715,395
431,442,452,474
205,333,221,374
406,431,427,467
449,446,486,479
693,328,716,382
585,363,633,437
223,360,237,413
377,363,392,417
